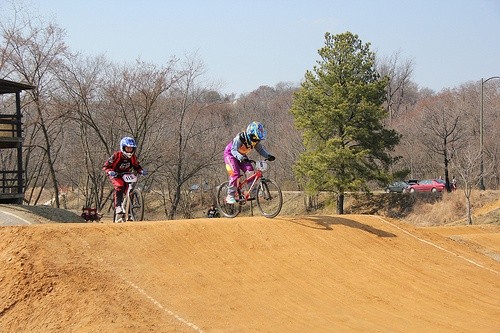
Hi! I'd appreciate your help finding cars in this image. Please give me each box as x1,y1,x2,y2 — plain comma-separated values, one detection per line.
384,179,454,193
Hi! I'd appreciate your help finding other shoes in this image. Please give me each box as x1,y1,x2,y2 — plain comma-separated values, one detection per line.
251,190,262,198
116,206,122,214
226,195,236,204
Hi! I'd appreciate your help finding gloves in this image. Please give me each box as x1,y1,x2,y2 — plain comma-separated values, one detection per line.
108,171,116,176
241,157,249,163
268,155,276,161
140,171,147,177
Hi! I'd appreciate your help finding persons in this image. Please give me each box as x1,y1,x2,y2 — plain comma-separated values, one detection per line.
102,137,147,214
439,175,444,180
207,204,216,218
223,121,275,204
452,175,457,192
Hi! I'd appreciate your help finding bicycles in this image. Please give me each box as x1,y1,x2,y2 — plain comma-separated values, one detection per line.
106,172,148,223
217,158,283,218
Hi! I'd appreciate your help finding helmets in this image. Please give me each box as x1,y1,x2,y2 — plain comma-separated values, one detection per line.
120,136,136,158
247,121,267,147
